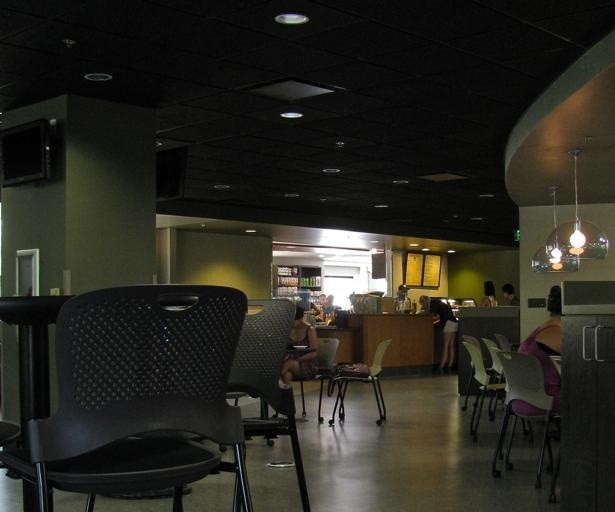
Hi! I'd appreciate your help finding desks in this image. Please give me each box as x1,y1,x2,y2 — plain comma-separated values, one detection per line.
0,294,75,512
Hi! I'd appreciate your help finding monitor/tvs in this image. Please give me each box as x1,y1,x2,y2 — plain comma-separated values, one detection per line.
0,118,49,187
156,146,188,202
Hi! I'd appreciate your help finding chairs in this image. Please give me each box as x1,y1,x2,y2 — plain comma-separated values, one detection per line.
179,299,317,511
270,338,339,422
0,284,255,512
459,330,564,505
328,338,393,425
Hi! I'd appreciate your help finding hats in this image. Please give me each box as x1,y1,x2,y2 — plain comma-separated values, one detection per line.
398,284,411,291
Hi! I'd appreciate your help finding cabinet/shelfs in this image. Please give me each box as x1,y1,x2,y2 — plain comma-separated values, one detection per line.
301,265,323,298
272,264,301,298
558,278,615,511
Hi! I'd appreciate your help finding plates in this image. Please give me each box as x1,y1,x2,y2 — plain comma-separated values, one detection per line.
316,322,325,325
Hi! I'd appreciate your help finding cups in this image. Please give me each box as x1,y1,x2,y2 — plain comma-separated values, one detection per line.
61,269,72,296
302,311,317,328
48,288,63,296
324,309,361,328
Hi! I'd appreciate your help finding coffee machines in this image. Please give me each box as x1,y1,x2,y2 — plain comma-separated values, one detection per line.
349,293,383,316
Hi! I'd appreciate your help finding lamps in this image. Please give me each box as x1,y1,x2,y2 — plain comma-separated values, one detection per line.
546,143,609,261
531,183,582,273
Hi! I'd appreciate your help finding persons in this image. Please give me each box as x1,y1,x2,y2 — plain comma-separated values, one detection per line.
419,295,459,372
394,285,411,310
512,285,561,416
296,289,320,321
502,283,520,306
280,306,319,387
322,294,342,320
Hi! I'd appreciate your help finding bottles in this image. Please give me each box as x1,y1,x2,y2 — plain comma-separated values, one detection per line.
395,285,410,317
277,267,321,296
412,299,417,314
